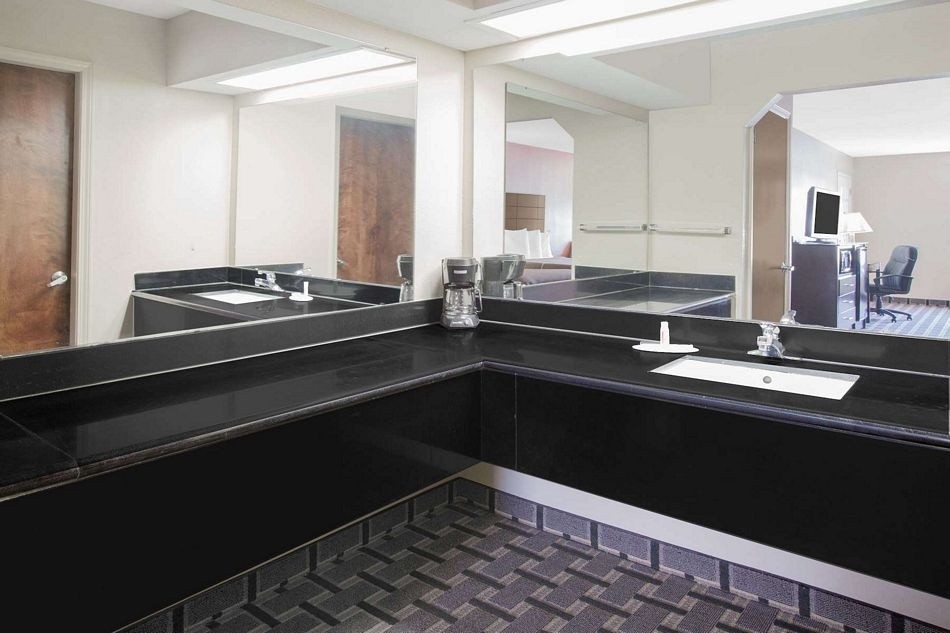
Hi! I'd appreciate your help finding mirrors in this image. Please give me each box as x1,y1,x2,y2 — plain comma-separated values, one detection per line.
503,81,649,286
0,0,419,359
234,82,414,288
472,0,950,344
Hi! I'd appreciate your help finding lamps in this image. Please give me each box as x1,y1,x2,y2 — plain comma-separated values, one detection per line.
843,212,873,243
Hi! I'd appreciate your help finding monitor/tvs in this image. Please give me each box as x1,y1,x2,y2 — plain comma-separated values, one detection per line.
807,185,843,239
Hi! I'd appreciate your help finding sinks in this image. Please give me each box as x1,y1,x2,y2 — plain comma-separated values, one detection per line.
650,354,861,402
193,289,285,306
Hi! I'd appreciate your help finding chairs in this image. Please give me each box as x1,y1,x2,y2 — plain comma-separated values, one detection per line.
868,245,917,323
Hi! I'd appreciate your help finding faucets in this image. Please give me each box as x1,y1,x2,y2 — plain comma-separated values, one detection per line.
295,268,312,274
254,270,282,291
756,323,786,361
780,309,798,325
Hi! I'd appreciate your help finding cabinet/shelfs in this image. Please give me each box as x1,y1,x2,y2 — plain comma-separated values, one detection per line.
791,242,872,329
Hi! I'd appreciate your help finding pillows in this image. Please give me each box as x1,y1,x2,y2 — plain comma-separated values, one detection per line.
504,228,553,260
